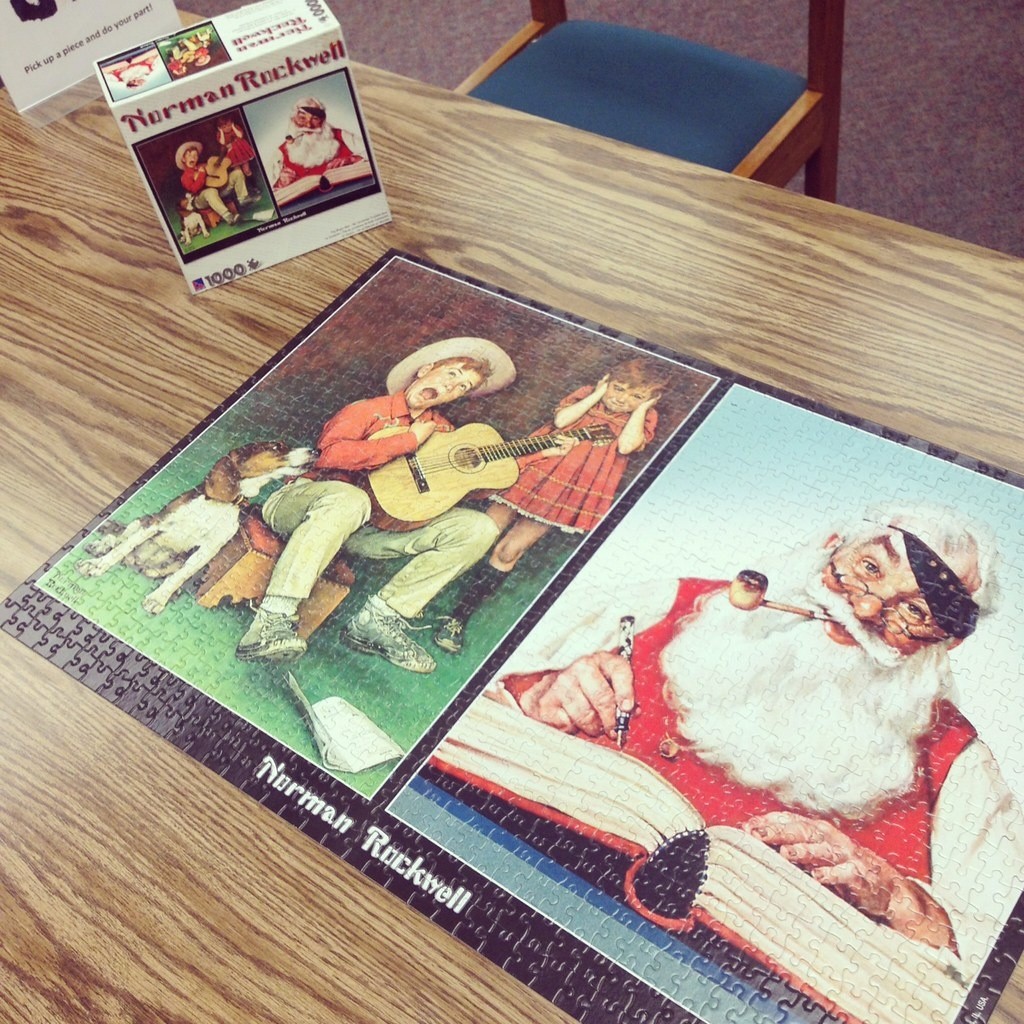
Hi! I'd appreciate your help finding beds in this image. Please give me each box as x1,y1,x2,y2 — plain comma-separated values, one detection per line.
452,0,844,206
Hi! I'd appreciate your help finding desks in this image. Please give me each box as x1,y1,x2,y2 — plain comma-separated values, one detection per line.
0,0,1024,1024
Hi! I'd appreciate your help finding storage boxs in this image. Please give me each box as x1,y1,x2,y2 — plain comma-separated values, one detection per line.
94,0,393,294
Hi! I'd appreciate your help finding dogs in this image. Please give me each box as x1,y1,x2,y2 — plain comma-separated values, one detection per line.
176,192,210,246
73,440,322,617
